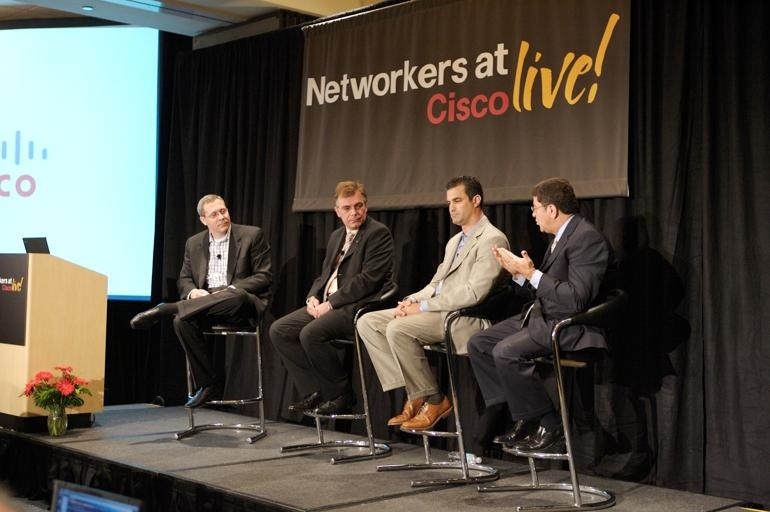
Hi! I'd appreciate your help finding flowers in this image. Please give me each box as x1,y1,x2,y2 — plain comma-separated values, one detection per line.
18,366,93,435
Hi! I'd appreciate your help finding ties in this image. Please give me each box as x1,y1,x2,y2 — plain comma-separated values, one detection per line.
323,233,355,302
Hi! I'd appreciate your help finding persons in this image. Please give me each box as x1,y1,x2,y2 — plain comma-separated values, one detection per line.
467,175,618,453
128,194,272,407
268,181,394,414
355,175,511,432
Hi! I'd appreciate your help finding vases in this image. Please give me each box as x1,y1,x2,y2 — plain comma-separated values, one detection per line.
47,404,68,437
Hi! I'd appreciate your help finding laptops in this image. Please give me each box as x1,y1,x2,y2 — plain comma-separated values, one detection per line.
23,238,50,253
50,479,145,512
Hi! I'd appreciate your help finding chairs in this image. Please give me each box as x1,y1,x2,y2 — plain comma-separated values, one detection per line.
174,315,268,444
476,287,625,512
377,272,521,488
280,278,400,465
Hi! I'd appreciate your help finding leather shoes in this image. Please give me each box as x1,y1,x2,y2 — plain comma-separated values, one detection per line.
387,394,453,430
289,389,357,415
185,384,220,408
130,302,164,329
493,420,565,453
